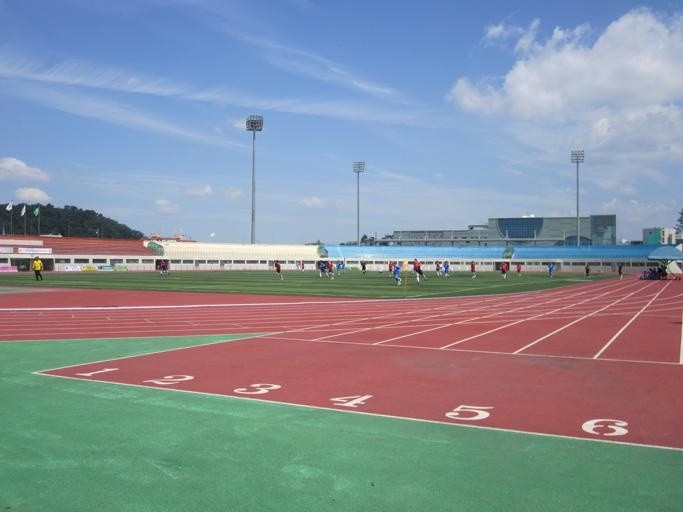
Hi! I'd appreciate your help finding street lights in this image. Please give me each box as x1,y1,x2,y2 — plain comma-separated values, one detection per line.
353,162,365,246
571,150,584,246
246,115,263,244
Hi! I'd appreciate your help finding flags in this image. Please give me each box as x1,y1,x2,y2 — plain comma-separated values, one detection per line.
33,205,40,217
5,201,12,211
20,204,27,217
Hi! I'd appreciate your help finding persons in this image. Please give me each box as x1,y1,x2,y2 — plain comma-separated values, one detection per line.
618,263,625,281
470,261,477,280
585,262,590,276
318,259,450,286
649,264,667,280
302,262,304,272
274,260,284,280
501,262,508,280
159,258,169,278
32,257,44,281
517,263,522,273
549,262,554,278
